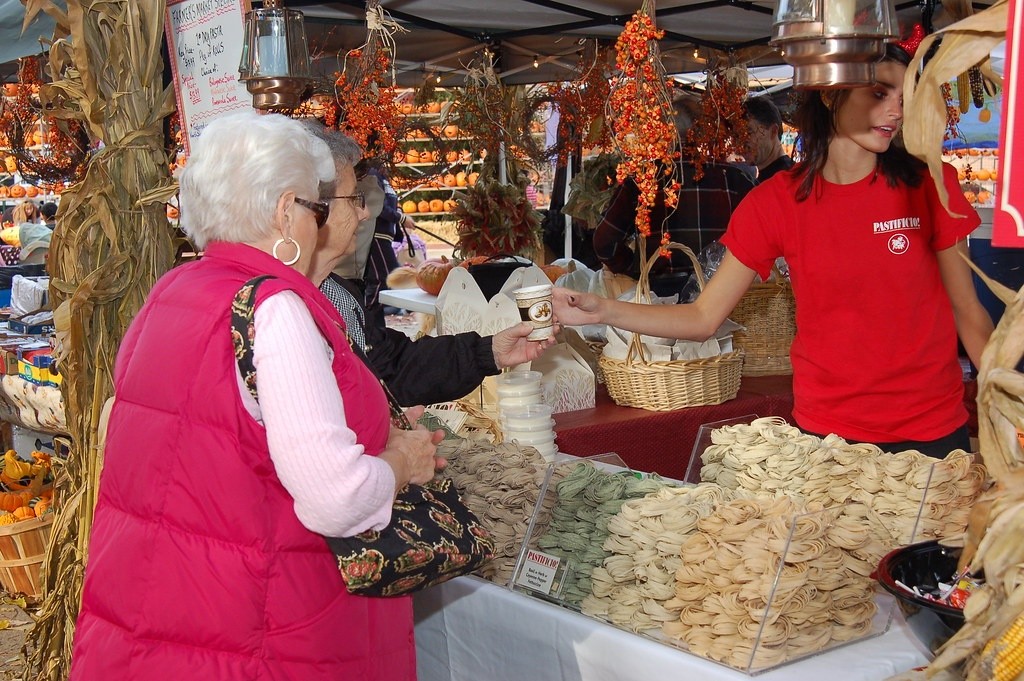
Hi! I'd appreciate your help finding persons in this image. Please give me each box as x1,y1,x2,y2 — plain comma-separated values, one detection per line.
67,111,559,681
549,46,1004,459
0,198,59,231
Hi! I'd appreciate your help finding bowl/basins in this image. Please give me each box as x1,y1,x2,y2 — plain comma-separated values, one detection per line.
877,538,990,672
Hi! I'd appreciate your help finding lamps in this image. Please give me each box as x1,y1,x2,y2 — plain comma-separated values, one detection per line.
768,0,901,91
238,0,314,109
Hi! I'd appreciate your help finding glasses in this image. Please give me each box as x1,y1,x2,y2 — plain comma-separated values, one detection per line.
318,190,365,210
295,198,329,229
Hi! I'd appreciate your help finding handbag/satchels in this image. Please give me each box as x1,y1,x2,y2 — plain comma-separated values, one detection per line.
229,277,497,601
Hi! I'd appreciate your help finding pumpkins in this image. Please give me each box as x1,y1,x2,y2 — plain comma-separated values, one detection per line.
417,253,637,300
978,108,991,121
947,147,999,203
0,448,59,526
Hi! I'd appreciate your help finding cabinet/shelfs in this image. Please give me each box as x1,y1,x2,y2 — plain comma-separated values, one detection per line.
391,90,507,220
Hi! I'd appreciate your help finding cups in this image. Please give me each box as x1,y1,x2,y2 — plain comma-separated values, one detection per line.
512,285,554,342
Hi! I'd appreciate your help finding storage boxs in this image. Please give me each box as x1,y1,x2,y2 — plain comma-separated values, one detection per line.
0,277,62,388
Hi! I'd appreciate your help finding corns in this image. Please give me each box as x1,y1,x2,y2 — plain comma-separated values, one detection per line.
957,64,984,114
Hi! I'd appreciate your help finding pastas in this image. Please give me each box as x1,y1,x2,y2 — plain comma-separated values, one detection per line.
426,414,992,675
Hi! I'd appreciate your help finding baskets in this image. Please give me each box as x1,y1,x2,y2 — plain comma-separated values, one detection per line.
728,266,798,379
598,241,744,413
